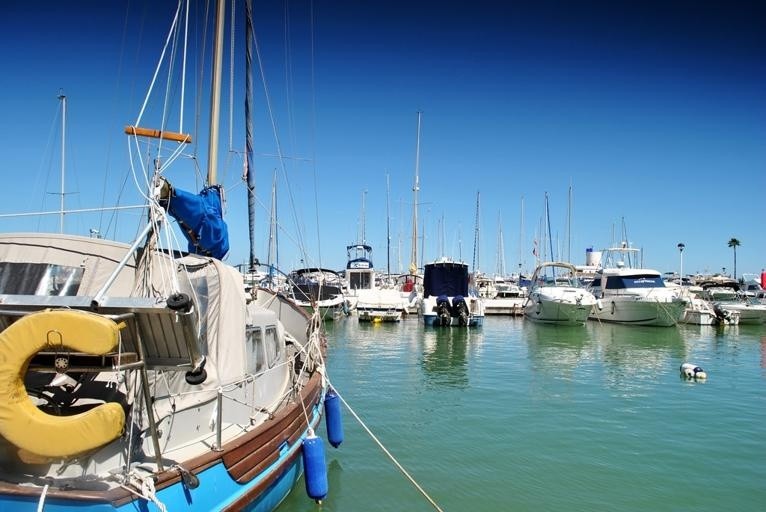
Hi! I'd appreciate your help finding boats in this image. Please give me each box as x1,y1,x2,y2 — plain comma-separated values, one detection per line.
659,271,765,326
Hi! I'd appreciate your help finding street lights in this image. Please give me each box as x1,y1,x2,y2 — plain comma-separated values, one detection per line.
677,244,684,286
